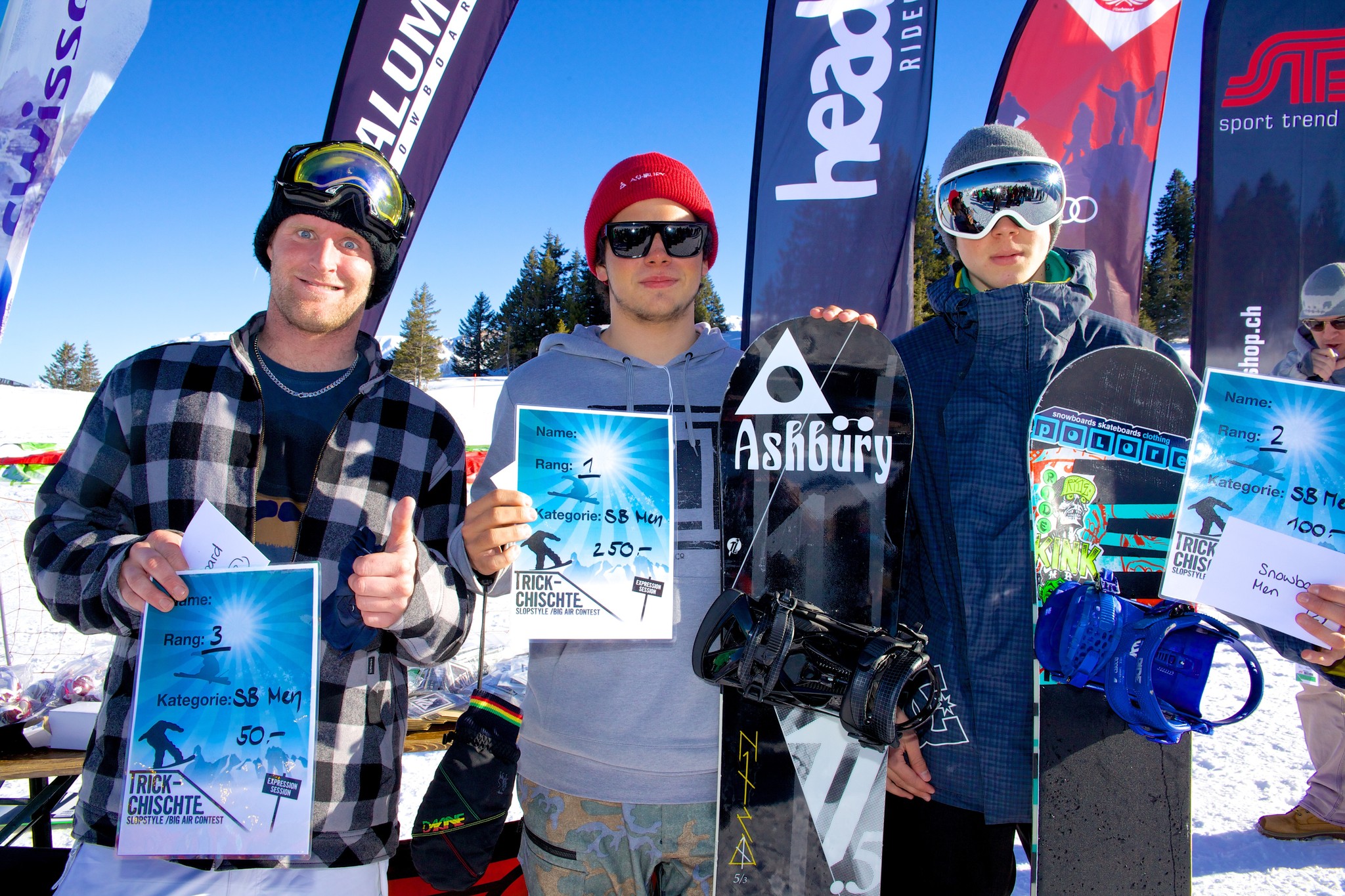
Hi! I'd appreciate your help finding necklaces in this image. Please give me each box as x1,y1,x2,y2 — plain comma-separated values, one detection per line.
254,331,360,398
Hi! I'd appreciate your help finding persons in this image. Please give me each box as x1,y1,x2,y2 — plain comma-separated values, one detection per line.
949,184,1049,235
1258,261,1345,840
877,125,1345,896
446,152,877,896
24,138,468,896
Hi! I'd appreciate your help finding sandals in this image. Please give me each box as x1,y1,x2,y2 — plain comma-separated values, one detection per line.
1035,569,1265,745
690,589,942,753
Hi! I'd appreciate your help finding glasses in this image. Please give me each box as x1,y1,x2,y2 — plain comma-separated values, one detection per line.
274,141,415,249
600,222,708,258
959,192,963,200
935,156,1066,239
1302,318,1345,331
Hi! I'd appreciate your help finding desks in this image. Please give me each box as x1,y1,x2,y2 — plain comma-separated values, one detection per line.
0,724,456,847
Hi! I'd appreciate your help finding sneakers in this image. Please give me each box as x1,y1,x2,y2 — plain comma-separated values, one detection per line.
1257,805,1345,841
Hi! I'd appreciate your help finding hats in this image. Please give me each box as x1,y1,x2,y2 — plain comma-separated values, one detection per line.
253,195,398,311
935,123,1062,260
1299,262,1345,320
584,152,718,286
949,190,959,210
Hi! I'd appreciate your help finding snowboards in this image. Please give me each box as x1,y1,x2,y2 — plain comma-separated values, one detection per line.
712,316,915,896
1025,346,1198,896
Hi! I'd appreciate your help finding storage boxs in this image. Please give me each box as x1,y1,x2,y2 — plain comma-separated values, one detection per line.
49,700,103,750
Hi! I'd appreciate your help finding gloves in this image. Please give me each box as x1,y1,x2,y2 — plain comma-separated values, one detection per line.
411,689,524,892
